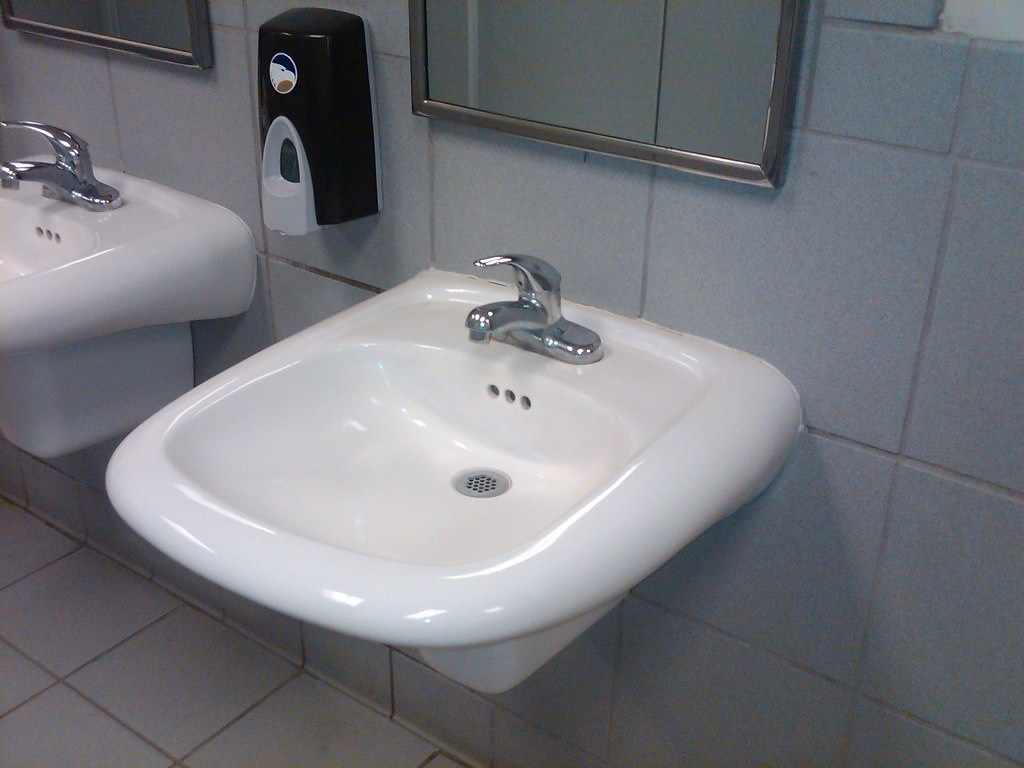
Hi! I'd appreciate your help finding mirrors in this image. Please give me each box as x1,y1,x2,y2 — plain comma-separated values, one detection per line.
0,0,217,72
406,2,808,194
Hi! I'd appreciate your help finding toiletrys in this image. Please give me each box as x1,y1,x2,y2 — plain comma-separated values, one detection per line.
252,6,386,242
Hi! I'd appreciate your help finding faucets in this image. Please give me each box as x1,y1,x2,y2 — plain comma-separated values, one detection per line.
0,117,120,210
462,249,605,360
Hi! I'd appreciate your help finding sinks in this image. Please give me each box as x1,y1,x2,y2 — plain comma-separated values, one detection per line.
101,262,806,698
0,152,260,460
